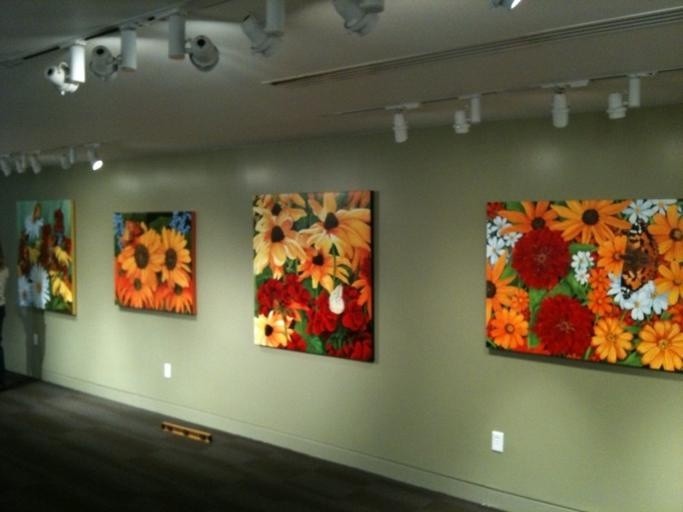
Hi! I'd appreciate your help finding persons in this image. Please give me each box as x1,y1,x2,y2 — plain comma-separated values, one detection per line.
0,240,9,391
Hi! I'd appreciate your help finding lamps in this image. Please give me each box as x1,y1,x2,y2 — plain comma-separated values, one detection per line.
0,144,103,181
48,1,389,96
391,74,644,146
492,0,522,11
